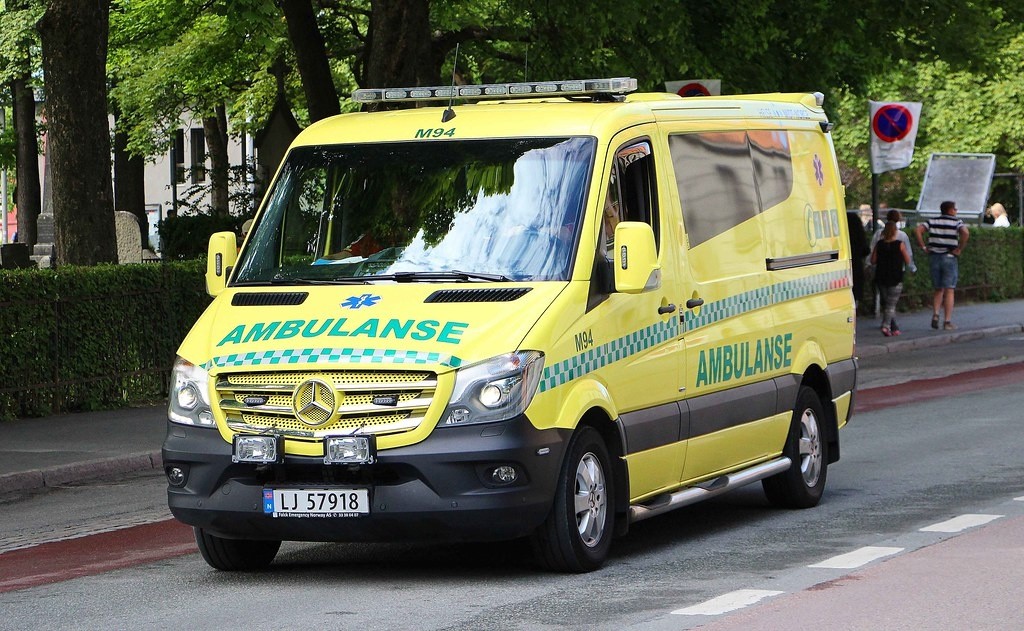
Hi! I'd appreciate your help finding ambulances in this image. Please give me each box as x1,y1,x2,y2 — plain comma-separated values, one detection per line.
162,77,858,571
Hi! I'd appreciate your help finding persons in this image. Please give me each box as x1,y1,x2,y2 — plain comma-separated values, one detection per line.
914,202,969,330
866,210,917,337
983,203,1010,227
322,185,412,260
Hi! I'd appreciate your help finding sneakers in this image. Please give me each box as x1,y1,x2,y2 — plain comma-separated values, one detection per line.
892,328,902,336
944,320,958,330
932,315,940,329
881,326,891,337
